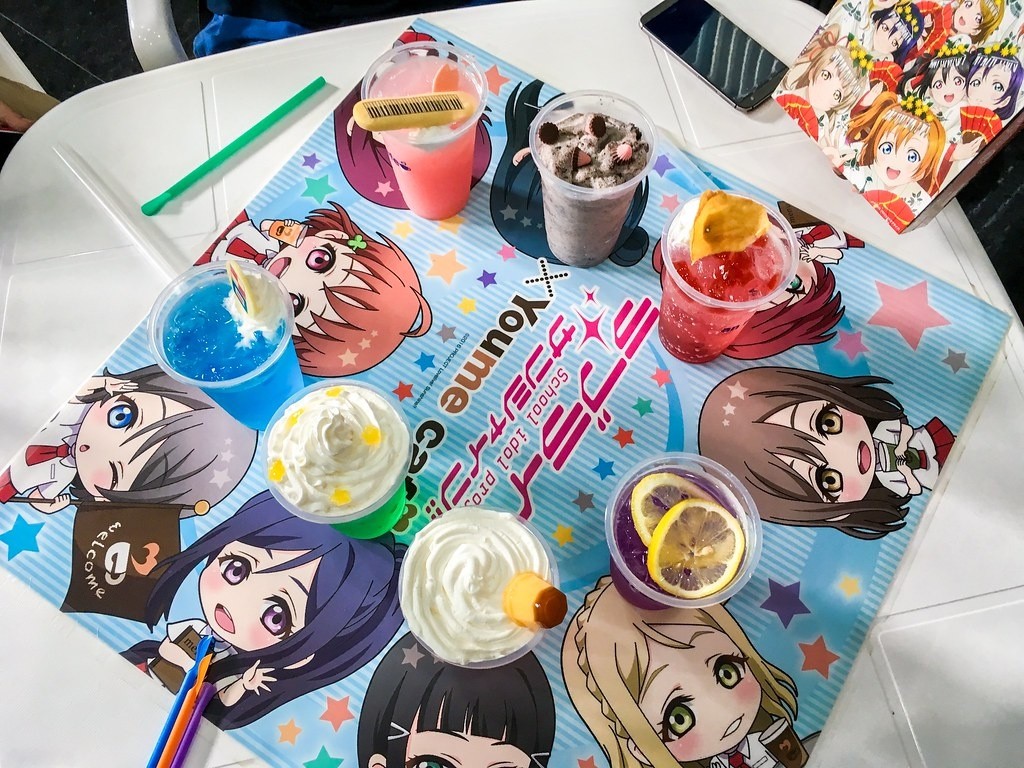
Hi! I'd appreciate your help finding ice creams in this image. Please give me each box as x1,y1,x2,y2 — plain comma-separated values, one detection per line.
532,112,650,269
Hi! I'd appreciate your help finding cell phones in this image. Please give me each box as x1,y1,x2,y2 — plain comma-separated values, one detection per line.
638,0,789,114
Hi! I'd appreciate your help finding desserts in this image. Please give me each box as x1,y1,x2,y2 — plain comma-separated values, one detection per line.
267,384,410,518
219,259,288,340
399,507,569,664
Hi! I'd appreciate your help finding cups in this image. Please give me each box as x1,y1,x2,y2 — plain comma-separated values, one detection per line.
659,190,798,364
605,452,762,610
528,88,660,267
360,43,488,220
257,377,414,537
149,260,305,431
396,505,566,669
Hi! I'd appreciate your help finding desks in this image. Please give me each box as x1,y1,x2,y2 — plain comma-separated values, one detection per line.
0,0,1024,768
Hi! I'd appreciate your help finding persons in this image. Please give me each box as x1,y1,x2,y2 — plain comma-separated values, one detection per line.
0,75,66,174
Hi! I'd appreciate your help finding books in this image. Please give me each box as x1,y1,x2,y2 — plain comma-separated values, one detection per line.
771,0,1024,235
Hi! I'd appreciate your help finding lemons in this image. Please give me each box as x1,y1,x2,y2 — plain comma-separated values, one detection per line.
631,472,745,598
